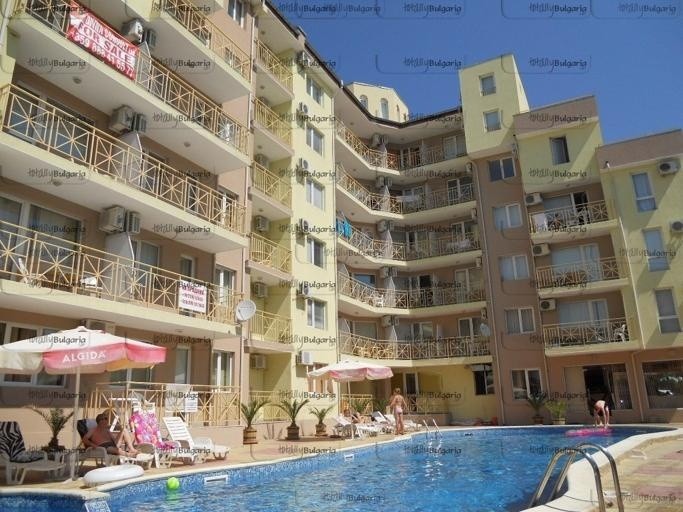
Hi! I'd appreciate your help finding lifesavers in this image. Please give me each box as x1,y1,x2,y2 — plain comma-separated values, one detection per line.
83,463,147,488
567,428,614,437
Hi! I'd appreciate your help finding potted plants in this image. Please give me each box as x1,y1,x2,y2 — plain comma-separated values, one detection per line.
525,391,548,425
267,399,310,440
308,406,333,436
546,400,569,425
233,398,272,445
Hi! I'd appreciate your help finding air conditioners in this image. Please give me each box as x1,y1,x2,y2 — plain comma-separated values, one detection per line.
669,218,683,233
251,50,313,369
98,18,157,235
80,318,116,335
657,160,678,177
370,133,400,328
466,163,556,320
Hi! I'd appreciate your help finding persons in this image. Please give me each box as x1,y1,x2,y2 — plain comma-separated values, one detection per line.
589,400,610,430
82,413,142,460
352,412,371,424
390,388,410,436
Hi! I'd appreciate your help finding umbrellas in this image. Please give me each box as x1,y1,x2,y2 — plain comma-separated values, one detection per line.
308,356,393,439
0,323,167,478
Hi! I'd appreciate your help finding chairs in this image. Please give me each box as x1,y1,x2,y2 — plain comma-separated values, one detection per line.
0,416,231,485
332,411,422,440
